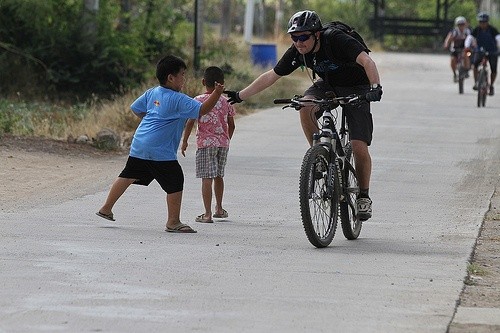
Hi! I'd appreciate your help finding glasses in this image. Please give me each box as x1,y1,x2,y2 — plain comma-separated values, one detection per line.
457,23,465,26
479,20,488,23
291,33,311,43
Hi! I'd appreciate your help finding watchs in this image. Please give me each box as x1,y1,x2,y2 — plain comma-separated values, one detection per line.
372,84,380,89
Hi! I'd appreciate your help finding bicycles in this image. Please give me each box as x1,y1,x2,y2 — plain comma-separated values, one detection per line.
439,46,477,95
273,91,382,249
464,46,500,108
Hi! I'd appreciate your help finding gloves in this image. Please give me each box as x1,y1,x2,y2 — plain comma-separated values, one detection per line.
223,90,245,105
366,88,383,102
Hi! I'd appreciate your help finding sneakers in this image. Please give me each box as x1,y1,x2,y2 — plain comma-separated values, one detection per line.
473,83,478,90
489,86,494,96
356,194,372,218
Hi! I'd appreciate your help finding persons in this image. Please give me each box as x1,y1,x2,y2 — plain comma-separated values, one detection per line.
465,11,500,94
223,10,383,219
95,56,225,233
181,66,235,223
446,16,471,81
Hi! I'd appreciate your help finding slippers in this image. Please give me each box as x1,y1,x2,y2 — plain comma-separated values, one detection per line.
165,224,198,233
195,214,214,223
213,208,229,219
96,210,117,221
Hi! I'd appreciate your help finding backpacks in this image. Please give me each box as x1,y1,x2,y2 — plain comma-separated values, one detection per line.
321,21,372,56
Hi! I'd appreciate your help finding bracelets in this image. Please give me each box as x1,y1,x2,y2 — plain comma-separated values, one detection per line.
235,92,241,102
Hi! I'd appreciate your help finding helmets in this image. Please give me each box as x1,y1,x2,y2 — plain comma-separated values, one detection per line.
286,10,323,33
478,13,489,21
455,17,466,24
